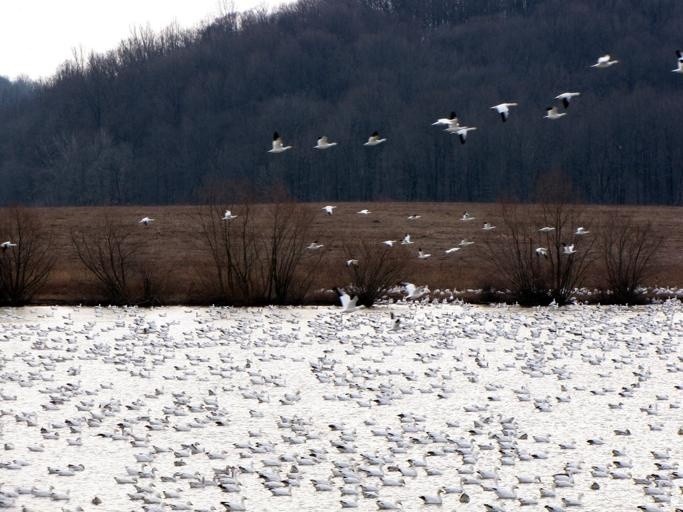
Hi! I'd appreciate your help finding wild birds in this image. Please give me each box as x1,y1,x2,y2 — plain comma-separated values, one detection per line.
671,53,683,73
432,111,460,129
0,281,683,512
220,207,238,221
267,131,292,154
544,105,567,120
442,125,477,143
364,131,386,146
138,216,154,230
305,203,591,270
314,134,337,149
555,93,580,108
0,240,18,249
489,102,518,122
591,55,618,68
